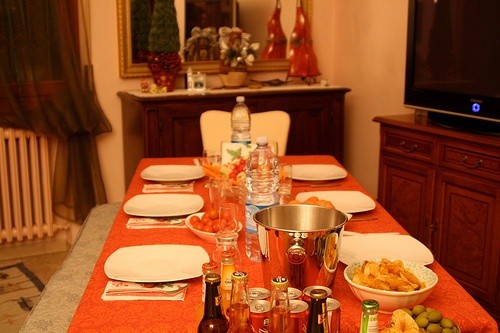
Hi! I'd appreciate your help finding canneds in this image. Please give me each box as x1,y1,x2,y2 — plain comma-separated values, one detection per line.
241,286,341,333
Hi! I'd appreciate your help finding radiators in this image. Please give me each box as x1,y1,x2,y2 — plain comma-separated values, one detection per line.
1,128,67,240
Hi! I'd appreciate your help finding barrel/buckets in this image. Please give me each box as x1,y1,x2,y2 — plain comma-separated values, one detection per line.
252,204,349,292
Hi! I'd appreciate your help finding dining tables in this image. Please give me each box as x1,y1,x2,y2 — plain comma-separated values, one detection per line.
66,156,499,333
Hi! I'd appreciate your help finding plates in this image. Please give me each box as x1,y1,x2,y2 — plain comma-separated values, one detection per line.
295,190,376,213
339,234,434,267
283,164,347,181
140,164,207,182
123,193,205,217
103,244,209,283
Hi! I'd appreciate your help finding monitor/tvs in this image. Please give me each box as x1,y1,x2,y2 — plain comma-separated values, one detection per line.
403,0,500,136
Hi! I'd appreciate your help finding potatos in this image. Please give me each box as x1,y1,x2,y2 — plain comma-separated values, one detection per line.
352,258,424,291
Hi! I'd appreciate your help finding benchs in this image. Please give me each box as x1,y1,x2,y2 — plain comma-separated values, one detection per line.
19,200,121,333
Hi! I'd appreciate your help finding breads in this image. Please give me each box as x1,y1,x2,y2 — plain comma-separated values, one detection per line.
287,196,336,210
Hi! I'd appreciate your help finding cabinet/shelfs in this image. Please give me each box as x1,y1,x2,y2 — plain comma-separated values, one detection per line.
116,92,346,195
373,114,500,314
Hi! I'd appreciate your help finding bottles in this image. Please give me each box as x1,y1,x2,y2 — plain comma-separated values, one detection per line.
141,76,149,93
243,137,280,263
231,96,252,145
195,229,378,333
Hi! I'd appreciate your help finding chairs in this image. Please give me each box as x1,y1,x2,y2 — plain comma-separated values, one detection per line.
200,110,291,158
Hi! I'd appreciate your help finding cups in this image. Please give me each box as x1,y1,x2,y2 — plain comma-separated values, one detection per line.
210,161,292,232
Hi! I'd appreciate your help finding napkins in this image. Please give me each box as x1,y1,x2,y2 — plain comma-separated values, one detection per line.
101,280,188,301
142,183,195,193
126,216,188,229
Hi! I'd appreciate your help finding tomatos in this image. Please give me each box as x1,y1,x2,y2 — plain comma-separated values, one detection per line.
190,209,236,232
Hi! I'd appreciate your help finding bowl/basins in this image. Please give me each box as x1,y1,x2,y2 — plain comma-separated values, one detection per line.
185,212,243,244
343,257,439,315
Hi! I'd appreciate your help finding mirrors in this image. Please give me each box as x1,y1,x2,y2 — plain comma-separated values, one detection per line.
115,0,311,77
